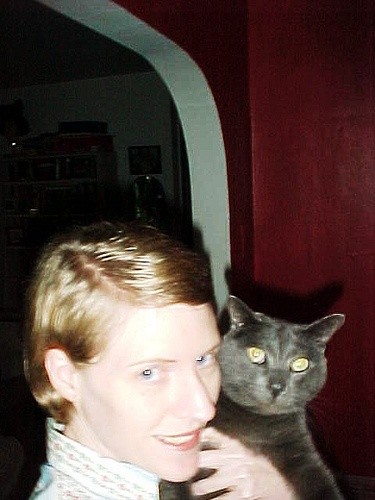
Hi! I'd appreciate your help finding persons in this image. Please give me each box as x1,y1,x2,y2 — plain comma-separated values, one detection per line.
23,220,296,500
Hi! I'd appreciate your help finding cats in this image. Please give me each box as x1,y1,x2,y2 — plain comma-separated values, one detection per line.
159,293,347,500
